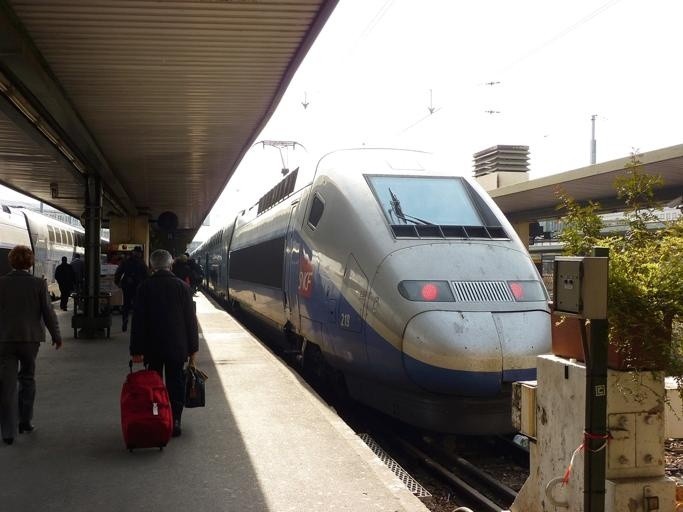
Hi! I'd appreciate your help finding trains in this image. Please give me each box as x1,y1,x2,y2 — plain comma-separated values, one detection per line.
191,146,557,438
1,201,110,300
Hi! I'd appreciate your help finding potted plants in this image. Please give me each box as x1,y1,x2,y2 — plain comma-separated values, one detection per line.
548,147,683,422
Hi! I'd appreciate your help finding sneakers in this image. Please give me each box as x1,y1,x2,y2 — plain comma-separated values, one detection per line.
2,421,35,446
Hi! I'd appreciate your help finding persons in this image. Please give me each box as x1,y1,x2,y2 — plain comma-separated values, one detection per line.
114,246,153,332
55,256,75,311
129,248,199,436
70,254,83,292
0,245,64,445
173,252,204,294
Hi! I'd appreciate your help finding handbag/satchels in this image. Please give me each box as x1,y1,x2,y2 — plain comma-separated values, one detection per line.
182,365,208,409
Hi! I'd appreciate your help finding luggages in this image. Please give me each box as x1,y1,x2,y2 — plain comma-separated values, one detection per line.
119,358,173,454
